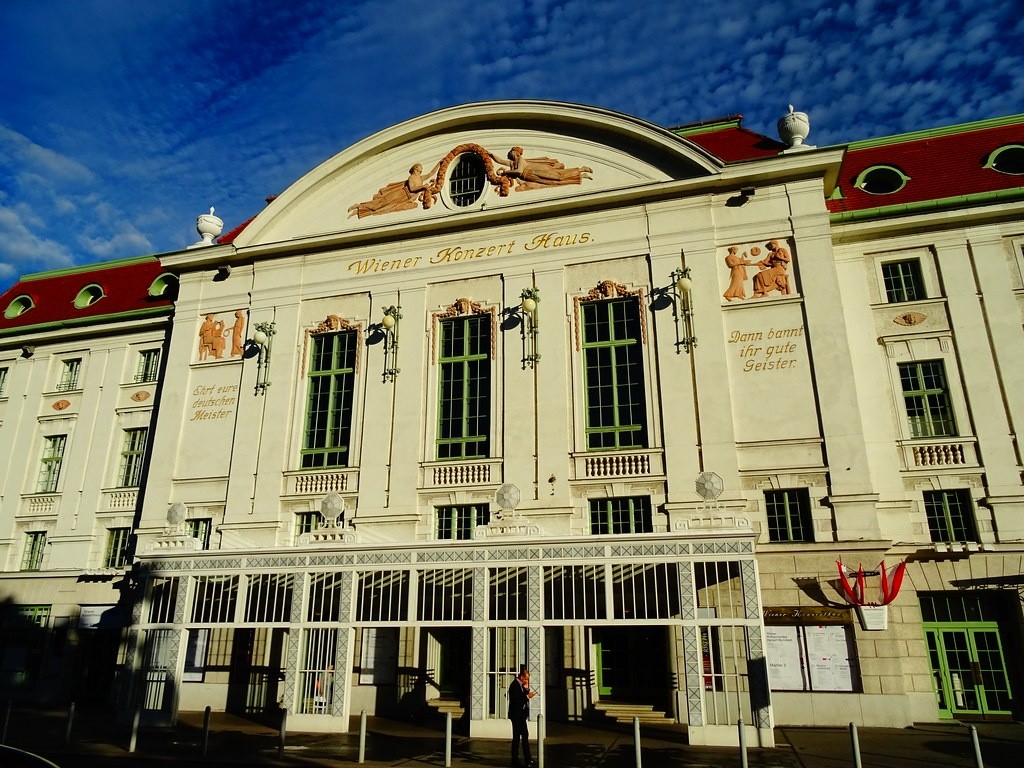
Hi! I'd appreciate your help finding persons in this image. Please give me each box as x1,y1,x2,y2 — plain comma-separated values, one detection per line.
508,669,535,768
200,310,245,358
347,145,593,221
724,240,791,301
314,665,335,709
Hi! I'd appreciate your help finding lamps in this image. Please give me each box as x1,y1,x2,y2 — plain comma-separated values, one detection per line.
965,542,979,552
81,568,119,576
950,542,963,552
675,266,697,350
982,542,994,551
253,321,277,395
383,305,400,379
935,542,948,553
520,287,541,367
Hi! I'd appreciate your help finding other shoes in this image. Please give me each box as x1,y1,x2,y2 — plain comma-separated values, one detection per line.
527,760,536,764
